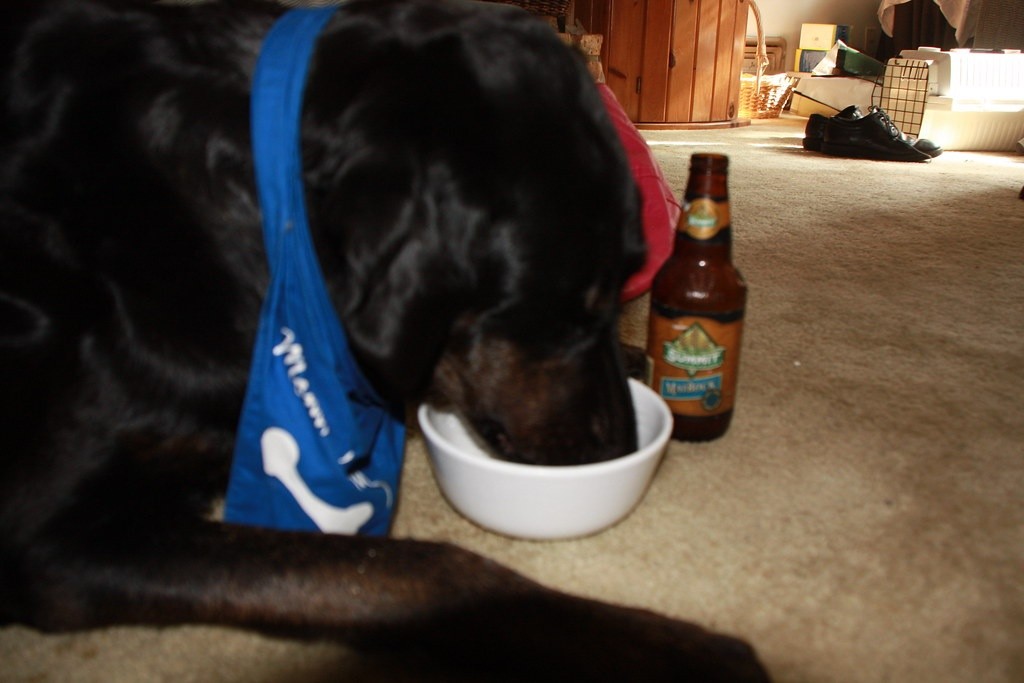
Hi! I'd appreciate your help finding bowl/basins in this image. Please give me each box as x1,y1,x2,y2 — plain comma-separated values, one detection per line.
416,376,675,540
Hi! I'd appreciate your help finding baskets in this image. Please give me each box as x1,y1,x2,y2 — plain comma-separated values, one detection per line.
738,0,801,118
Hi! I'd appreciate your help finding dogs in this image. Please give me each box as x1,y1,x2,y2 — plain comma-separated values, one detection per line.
0,0,779,683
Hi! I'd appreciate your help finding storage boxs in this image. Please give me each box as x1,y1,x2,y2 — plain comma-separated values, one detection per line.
798,22,851,50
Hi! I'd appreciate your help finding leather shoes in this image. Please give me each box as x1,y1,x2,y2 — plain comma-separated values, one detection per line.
803,105,943,163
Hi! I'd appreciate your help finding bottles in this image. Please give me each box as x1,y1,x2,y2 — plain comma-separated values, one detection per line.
646,154,747,444
